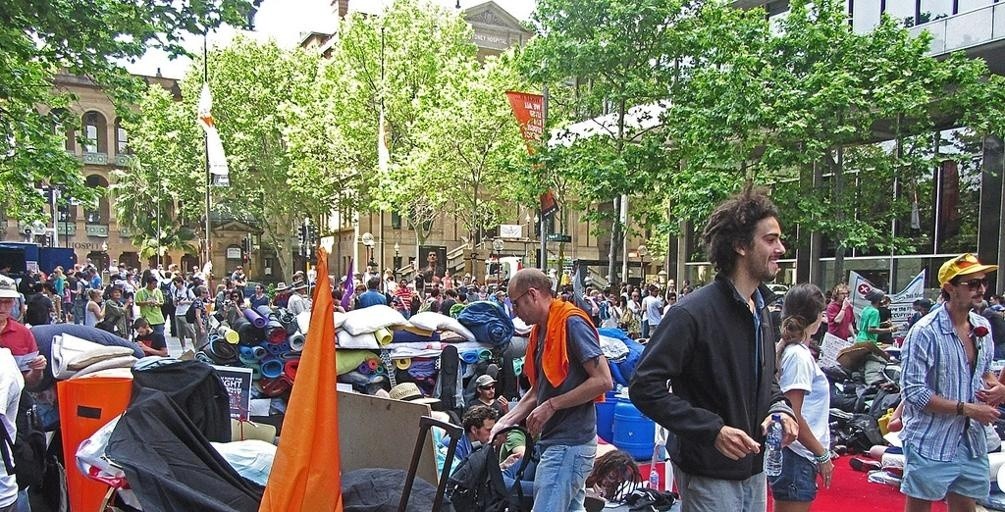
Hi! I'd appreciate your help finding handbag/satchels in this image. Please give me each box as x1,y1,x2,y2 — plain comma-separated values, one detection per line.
93,320,115,336
0,389,49,493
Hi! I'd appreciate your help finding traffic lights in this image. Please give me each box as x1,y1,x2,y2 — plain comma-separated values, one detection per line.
296,226,320,243
42,186,48,203
241,239,245,251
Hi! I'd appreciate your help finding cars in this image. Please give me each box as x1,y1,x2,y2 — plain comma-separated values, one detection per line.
764,281,788,336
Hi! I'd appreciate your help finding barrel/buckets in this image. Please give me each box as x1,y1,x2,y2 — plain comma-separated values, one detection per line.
595,390,622,444
612,397,655,462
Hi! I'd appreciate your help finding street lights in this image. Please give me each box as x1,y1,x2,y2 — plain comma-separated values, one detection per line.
638,243,649,286
394,242,400,269
492,239,504,287
362,232,374,270
525,211,530,242
370,240,376,264
533,213,539,239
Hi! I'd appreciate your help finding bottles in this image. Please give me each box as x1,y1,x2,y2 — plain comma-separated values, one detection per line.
648,469,660,491
763,412,785,478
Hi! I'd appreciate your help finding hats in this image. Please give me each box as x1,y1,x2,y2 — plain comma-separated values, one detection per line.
274,281,291,291
290,280,311,291
0,273,21,298
864,287,886,302
475,375,499,389
385,380,445,406
936,253,999,289
109,286,123,297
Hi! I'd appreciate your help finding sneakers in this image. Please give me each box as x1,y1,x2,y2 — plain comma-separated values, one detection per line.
849,456,882,473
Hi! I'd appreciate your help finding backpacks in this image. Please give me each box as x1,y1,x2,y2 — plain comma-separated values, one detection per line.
159,280,173,306
185,302,198,324
445,425,535,512
79,280,90,301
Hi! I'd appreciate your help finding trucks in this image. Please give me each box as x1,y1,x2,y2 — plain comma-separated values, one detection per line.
484,256,583,287
0,241,79,279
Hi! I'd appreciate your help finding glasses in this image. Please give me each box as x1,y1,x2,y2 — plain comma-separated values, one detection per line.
231,294,238,298
480,385,497,391
952,276,992,289
668,297,676,300
508,287,538,310
